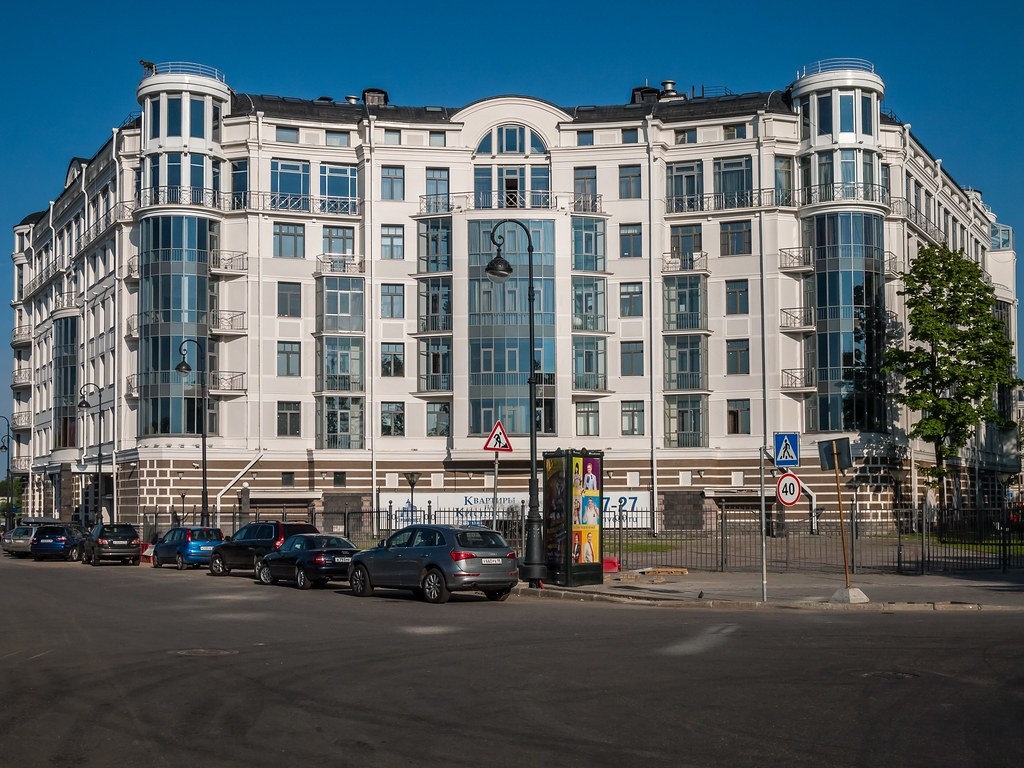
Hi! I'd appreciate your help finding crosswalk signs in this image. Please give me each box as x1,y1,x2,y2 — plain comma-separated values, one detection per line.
773,430,800,468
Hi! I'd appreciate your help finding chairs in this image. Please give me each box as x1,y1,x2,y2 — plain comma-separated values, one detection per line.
415,531,432,546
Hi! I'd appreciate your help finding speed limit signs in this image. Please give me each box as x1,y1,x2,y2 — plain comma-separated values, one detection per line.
776,472,801,507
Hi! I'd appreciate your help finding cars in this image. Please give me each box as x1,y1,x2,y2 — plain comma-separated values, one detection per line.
152,526,224,570
346,523,521,605
258,532,362,589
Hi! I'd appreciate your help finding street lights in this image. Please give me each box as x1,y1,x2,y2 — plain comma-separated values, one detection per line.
175,339,210,527
78,382,103,525
486,219,548,583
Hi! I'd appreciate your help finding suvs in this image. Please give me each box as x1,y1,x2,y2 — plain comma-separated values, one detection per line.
1,524,84,563
80,522,141,566
208,519,321,581
